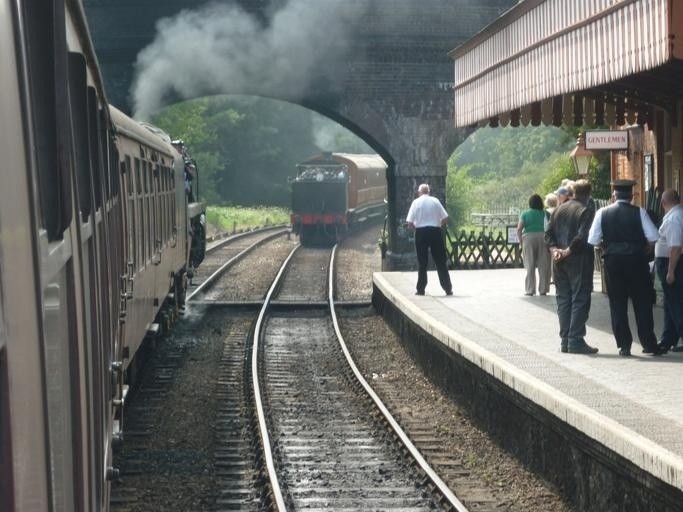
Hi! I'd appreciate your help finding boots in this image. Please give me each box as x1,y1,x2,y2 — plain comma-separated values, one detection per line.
561,337,599,354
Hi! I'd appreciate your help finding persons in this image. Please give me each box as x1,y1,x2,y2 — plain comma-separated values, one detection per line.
404,181,455,298
653,187,683,354
587,180,661,355
543,179,600,355
515,176,596,298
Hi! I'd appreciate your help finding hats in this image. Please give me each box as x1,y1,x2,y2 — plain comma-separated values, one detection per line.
609,179,637,191
554,187,569,196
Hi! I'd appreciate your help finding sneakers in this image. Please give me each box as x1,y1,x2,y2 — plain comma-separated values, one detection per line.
415,290,425,296
618,342,683,355
447,291,453,295
525,282,554,297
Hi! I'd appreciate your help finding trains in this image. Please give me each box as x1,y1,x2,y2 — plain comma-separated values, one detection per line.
0,1,204,510
287,148,389,250
